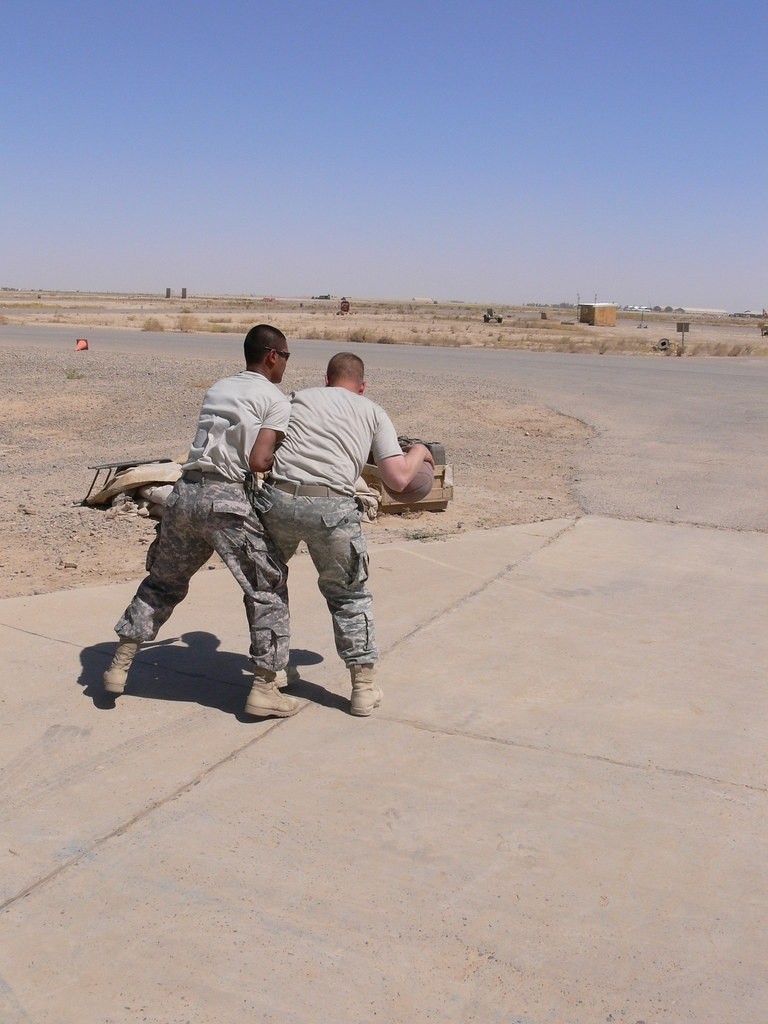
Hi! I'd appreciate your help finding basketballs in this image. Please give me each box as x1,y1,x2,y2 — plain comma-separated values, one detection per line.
380,451,434,504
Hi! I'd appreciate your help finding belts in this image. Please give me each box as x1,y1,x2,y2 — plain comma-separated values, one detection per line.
181,470,245,490
263,477,350,498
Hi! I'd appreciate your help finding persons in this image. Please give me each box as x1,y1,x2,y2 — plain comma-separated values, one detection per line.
102,324,301,716
243,352,435,715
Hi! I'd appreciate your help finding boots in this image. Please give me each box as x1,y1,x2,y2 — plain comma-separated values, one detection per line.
273,666,300,689
349,663,383,716
244,666,300,717
101,637,138,693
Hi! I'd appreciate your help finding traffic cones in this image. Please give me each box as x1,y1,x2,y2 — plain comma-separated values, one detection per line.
74,338,88,351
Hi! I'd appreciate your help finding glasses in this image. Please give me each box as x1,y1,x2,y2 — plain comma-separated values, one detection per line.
263,347,291,362
